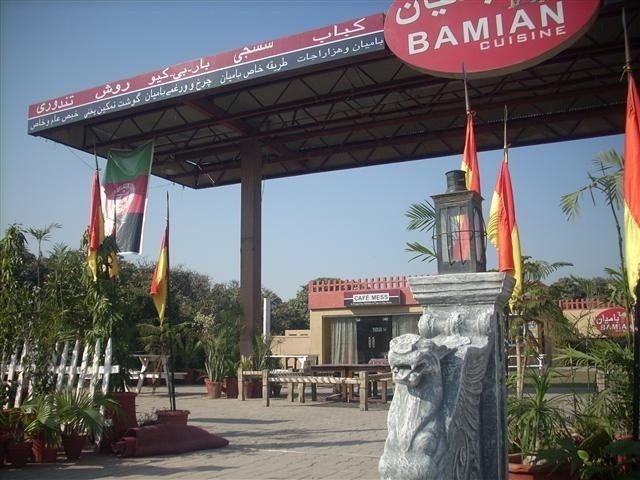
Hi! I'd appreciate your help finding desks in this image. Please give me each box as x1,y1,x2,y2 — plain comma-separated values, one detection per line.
310,364,390,403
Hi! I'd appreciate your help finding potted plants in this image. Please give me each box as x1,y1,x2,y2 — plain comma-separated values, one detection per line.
241,355,258,398
204,350,230,398
494,256,640,480
226,359,242,399
0,223,137,468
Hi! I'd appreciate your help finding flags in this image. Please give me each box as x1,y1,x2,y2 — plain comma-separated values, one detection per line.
448,112,488,264
150,217,169,326
87,167,106,285
621,72,640,308
98,138,156,254
484,158,526,313
105,224,120,290
